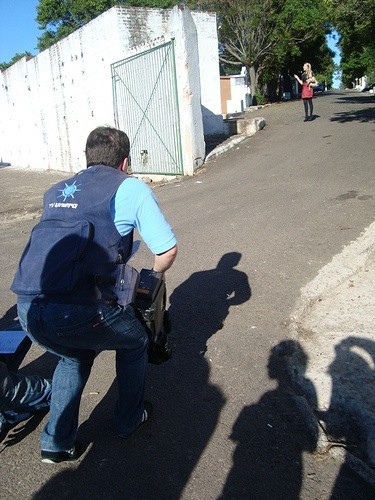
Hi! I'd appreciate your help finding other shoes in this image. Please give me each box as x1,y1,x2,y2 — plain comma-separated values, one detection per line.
120,400,152,440
41,448,79,464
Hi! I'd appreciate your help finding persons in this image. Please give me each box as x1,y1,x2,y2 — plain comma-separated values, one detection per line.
10,128,177,463
294,63,317,122
